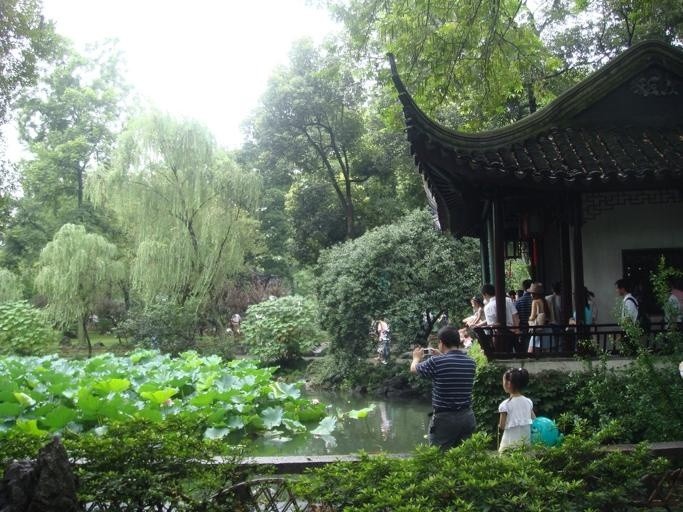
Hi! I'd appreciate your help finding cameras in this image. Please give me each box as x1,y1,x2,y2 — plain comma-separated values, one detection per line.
422,348,433,356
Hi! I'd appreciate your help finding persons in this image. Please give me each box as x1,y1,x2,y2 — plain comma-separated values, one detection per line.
373,318,393,363
660,277,682,355
407,324,478,453
377,401,393,443
610,277,642,357
455,272,598,359
496,365,537,458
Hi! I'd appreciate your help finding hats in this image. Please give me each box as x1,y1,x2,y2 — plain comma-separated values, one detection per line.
526,281,547,296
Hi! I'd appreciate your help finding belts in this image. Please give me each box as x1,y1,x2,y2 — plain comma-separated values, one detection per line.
427,405,471,418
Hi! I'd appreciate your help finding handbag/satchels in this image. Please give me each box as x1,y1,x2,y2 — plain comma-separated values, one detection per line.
527,312,546,327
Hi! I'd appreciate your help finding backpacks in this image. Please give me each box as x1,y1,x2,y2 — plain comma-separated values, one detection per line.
624,297,652,335
